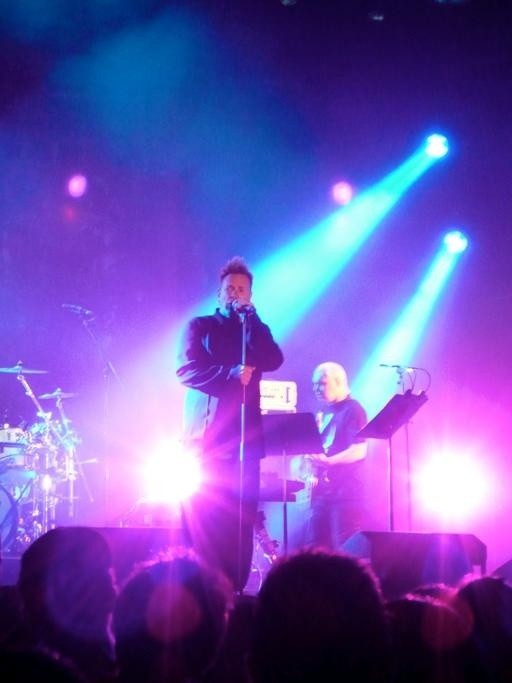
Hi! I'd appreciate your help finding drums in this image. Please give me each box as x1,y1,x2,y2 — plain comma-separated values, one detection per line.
0,427,37,482
0,486,19,557
30,444,68,478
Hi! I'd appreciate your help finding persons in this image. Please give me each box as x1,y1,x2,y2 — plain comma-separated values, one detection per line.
304,361,371,550
176,257,284,589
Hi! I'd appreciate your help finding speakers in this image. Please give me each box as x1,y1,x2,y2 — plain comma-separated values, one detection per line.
56,526,193,583
339,531,487,601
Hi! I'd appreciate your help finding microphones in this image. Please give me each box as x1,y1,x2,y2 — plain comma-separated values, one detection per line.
232,299,255,314
62,303,95,322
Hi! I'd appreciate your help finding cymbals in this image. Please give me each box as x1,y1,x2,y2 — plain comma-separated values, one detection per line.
39,392,77,400
0,367,47,375
